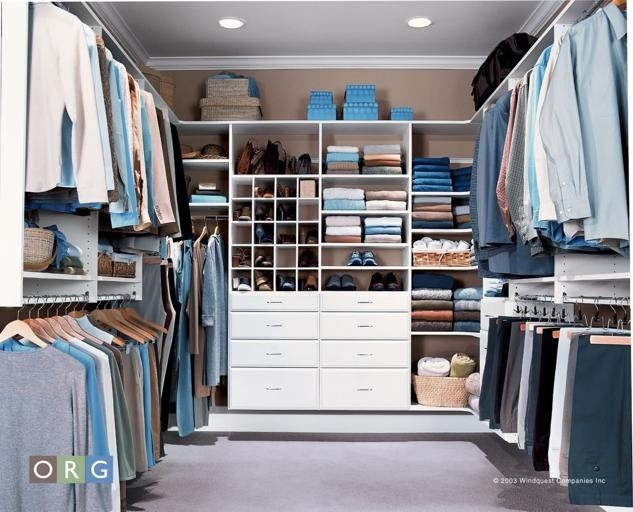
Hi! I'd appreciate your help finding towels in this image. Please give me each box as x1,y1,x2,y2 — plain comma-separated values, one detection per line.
417,351,481,416
412,236,472,252
61,255,86,274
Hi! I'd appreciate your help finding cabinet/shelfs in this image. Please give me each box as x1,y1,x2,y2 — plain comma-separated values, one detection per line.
472,0,631,510
409,120,482,414
2,2,174,509
177,119,230,416
227,293,412,412
230,121,412,292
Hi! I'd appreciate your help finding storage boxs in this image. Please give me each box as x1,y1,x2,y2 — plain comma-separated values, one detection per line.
96,244,137,279
200,75,263,121
304,82,415,120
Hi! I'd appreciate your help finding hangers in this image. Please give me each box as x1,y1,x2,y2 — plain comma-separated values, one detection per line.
610,0,626,12
0,291,169,353
185,214,222,247
494,290,631,345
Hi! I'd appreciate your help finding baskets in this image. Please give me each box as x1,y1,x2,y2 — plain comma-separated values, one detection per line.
98,250,136,278
197,97,266,121
140,62,162,93
159,75,177,116
24,227,58,272
204,77,252,97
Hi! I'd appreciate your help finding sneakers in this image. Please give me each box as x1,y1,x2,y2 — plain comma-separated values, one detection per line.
254,250,273,266
346,250,363,266
299,250,318,266
384,272,401,290
232,272,251,291
340,274,357,290
276,272,295,291
325,274,342,290
303,274,318,291
299,226,319,244
255,204,273,220
253,181,274,199
368,272,385,290
233,205,252,221
362,251,379,266
254,270,273,291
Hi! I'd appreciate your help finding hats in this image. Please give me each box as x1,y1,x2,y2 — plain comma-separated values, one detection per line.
179,142,200,158
199,143,229,160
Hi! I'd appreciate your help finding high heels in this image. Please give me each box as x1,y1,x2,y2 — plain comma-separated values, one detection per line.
254,223,274,244
276,204,295,220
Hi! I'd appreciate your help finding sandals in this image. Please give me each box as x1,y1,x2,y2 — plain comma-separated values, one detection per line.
232,247,250,267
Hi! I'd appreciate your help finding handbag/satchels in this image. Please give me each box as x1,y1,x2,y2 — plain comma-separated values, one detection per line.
264,139,287,174
296,153,312,173
287,156,298,173
470,31,539,113
237,137,256,174
250,147,266,174
